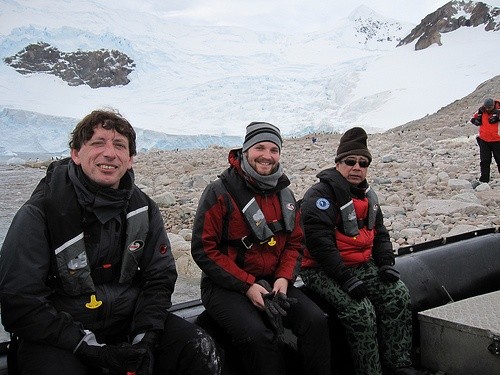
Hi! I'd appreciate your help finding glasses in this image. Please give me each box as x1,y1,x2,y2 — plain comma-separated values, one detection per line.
339,159,369,168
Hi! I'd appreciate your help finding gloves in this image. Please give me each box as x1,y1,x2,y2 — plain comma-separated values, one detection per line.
489,116,499,124
474,119,481,126
377,265,401,287
131,331,157,375
257,279,297,334
73,333,145,375
338,271,369,303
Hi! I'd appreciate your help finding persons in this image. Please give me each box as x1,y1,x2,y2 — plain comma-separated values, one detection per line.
0,111,222,375
470,98,500,183
299,127,423,375
191,122,332,375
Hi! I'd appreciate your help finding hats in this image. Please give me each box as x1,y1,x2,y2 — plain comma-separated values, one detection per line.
484,99,495,106
335,127,372,164
242,122,282,156
